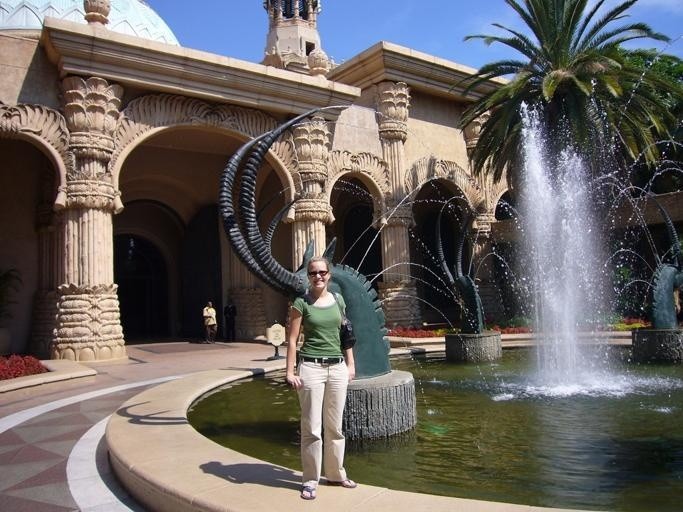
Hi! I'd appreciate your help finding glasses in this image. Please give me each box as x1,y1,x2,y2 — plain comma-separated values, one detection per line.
307,270,329,276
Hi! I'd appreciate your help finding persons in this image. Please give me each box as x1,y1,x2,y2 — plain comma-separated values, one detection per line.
224,299,237,342
283,256,357,499
202,301,217,344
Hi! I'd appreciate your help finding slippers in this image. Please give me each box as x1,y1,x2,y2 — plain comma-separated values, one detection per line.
301,485,315,499
329,479,357,489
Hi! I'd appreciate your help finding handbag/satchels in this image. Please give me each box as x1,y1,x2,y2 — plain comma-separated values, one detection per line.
341,319,357,351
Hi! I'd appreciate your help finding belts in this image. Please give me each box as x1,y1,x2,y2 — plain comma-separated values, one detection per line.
299,357,344,365
